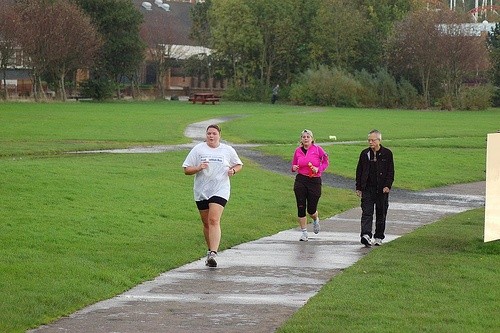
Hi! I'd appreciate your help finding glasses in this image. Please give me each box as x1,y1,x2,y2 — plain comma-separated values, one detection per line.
368,138,378,142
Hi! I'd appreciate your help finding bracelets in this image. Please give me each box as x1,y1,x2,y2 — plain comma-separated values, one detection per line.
231,168,235,174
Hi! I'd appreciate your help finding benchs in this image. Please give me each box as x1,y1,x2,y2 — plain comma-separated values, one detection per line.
204,98,220,101
188,98,201,102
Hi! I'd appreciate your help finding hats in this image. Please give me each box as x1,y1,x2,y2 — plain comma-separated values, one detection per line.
301,129,313,137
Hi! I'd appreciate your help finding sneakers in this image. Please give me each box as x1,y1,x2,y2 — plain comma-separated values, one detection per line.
205,251,217,267
299,232,308,241
374,238,382,246
361,234,372,246
313,219,319,234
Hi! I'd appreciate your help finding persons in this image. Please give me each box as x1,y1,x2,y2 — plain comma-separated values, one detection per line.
291,129,329,242
271,84,280,104
182,125,243,268
356,129,395,248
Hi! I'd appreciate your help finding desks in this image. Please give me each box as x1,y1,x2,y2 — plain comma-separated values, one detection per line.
191,93,216,105
